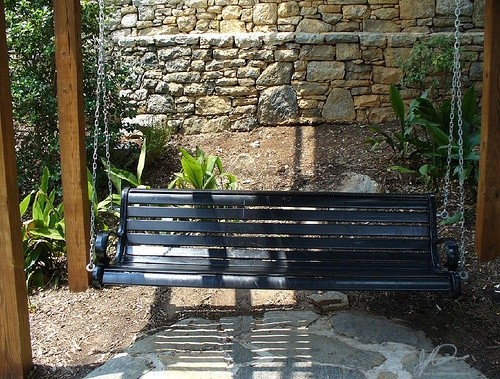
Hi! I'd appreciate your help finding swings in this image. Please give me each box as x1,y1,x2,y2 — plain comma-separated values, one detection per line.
90,1,471,299
